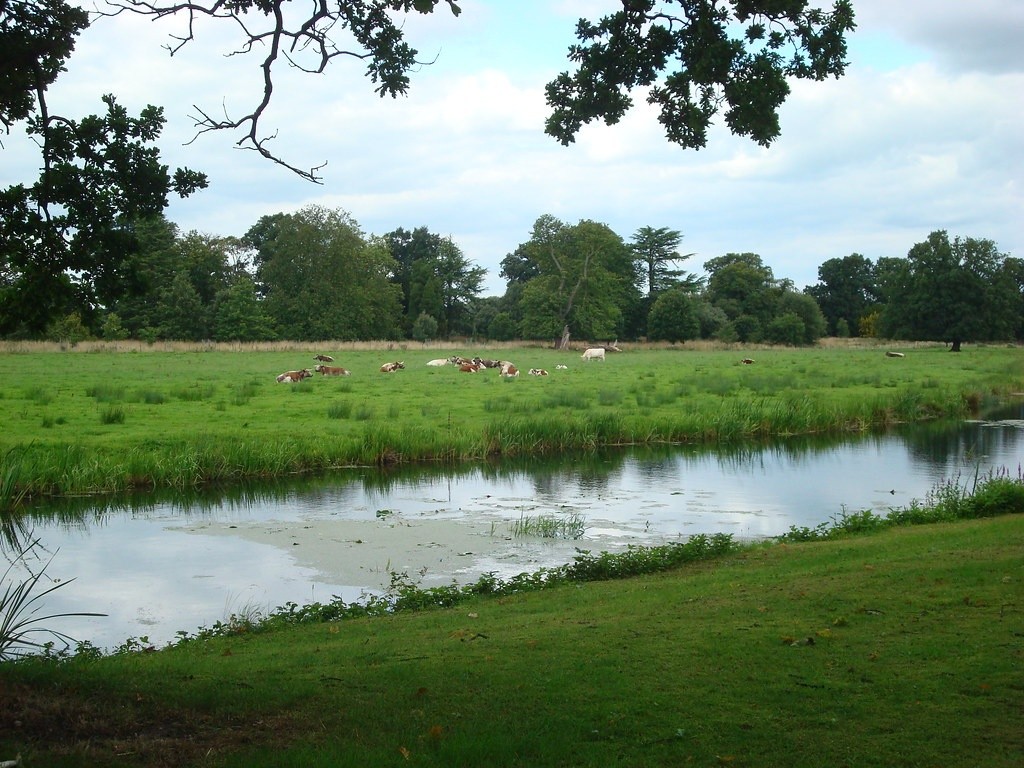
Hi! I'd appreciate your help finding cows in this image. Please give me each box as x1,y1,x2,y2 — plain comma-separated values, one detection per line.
741,359,755,364
313,364,352,378
528,368,548,376
276,368,313,384
426,356,520,378
378,360,405,372
580,348,606,362
886,351,904,357
313,354,334,362
